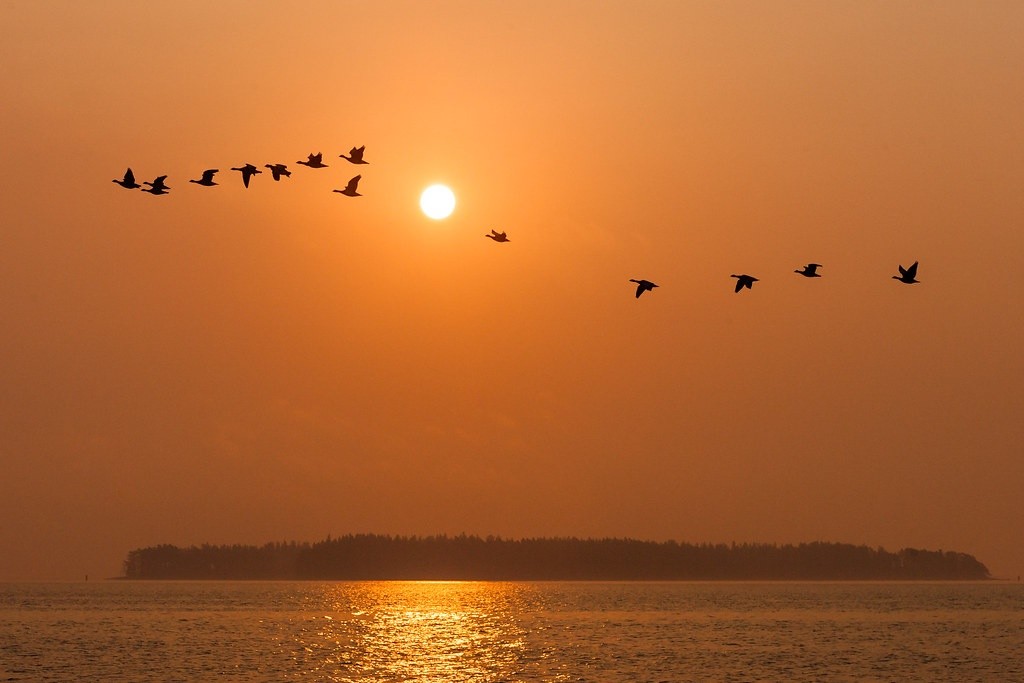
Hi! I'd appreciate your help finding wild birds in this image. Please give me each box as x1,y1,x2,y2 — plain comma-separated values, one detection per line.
891,261,923,284
794,264,825,278
264,164,291,182
296,152,328,170
485,229,512,243
332,174,363,198
230,163,263,188
729,273,761,293
339,146,370,165
629,279,661,299
111,168,171,195
189,168,220,187
